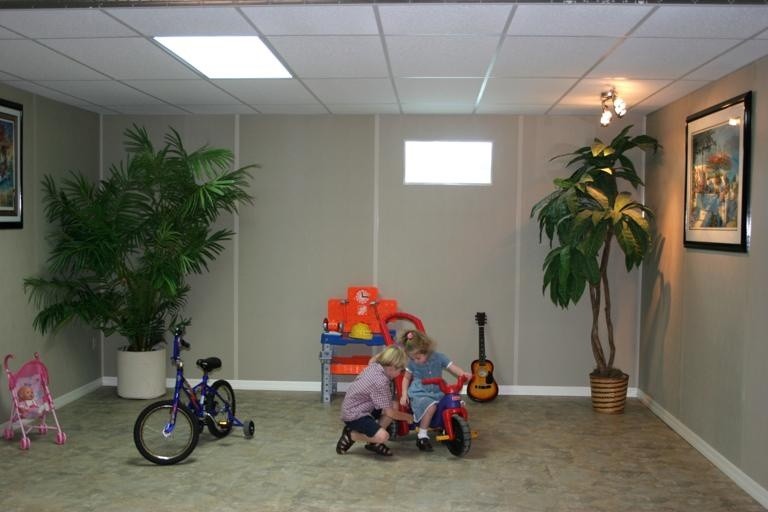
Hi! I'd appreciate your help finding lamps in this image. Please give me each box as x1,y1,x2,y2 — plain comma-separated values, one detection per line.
600,89,627,127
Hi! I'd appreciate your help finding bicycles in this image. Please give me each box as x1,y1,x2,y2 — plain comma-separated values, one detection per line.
133,322,255,466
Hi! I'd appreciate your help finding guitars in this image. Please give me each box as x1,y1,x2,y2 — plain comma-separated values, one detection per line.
467,314,498,402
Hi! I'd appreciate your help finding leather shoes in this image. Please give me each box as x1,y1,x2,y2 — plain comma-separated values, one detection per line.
416,436,432,451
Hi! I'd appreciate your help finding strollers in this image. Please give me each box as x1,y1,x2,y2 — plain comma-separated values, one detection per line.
3,349,67,451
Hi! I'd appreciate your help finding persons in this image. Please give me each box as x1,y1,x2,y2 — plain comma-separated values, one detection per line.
334,344,414,458
397,329,475,451
16,385,50,420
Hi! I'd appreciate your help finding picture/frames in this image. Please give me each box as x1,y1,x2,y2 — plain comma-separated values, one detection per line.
0,99,24,229
681,90,753,254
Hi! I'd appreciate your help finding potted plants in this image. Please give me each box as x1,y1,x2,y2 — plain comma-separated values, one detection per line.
530,125,663,414
24,120,261,399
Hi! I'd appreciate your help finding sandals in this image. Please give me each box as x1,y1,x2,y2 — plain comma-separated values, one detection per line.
336,426,355,454
365,442,393,456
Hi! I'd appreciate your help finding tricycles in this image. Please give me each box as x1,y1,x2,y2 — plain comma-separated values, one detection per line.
376,312,479,459
